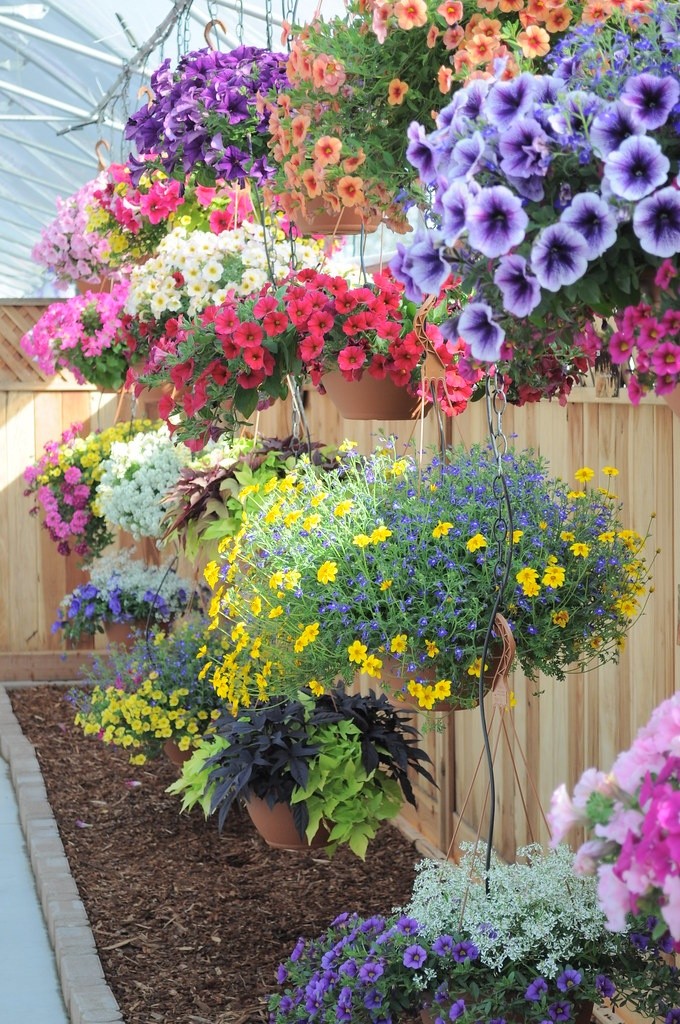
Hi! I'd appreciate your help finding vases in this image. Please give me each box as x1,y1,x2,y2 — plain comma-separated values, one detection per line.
242,788,335,849
322,369,434,422
127,355,169,403
162,742,206,765
418,994,524,1024
103,618,168,653
290,186,381,236
565,992,594,1024
372,648,505,709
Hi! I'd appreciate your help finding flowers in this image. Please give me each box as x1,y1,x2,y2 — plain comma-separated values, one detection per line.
20,1,680,1024
